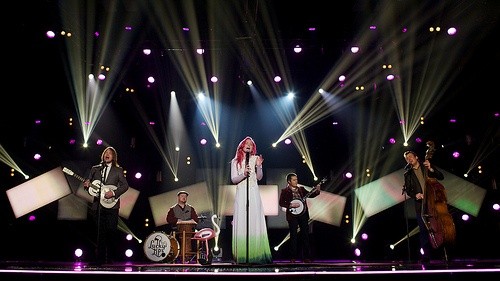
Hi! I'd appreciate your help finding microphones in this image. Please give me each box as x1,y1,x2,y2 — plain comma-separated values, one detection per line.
99,160,105,170
404,162,411,169
246,149,250,160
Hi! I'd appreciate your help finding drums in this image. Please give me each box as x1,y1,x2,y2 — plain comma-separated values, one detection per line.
143,231,179,264
178,220,197,258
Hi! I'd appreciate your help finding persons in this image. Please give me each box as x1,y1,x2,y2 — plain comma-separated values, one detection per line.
279,173,320,263
167,190,203,252
230,136,273,264
403,150,456,270
83,146,129,266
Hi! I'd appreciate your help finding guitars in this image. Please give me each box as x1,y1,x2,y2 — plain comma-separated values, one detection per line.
63,167,120,208
289,177,327,216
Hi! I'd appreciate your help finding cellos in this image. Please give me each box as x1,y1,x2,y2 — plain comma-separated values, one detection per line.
421,141,456,262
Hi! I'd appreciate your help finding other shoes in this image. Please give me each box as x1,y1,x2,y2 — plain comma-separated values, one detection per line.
303,258,312,263
290,258,295,263
